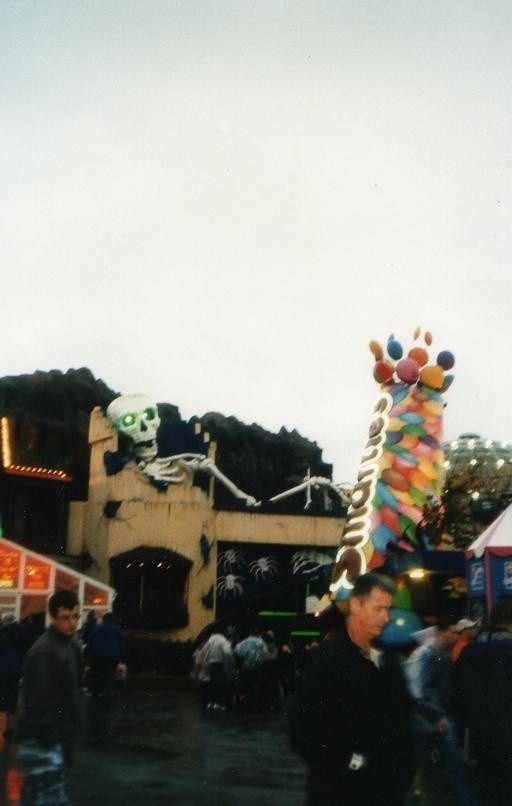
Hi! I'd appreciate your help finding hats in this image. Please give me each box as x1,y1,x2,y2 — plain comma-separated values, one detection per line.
452,618,481,632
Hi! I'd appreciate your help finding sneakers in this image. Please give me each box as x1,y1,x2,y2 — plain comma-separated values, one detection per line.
206,702,226,712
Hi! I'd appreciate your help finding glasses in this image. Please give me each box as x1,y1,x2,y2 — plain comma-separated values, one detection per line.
56,614,80,621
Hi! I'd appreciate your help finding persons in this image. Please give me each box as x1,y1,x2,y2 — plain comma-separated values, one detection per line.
10,588,88,806
280,570,415,806
0,607,48,715
85,613,125,704
